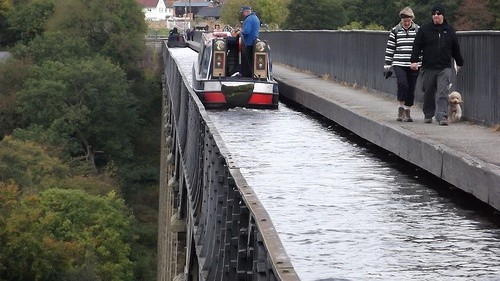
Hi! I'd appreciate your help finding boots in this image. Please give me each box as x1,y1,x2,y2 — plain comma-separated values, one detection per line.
397,107,405,121
404,109,413,122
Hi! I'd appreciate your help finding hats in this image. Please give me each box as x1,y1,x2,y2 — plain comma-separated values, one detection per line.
240,6,251,12
431,4,445,15
399,7,415,19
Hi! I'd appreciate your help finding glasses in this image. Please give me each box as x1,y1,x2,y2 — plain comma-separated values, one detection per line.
432,12,442,15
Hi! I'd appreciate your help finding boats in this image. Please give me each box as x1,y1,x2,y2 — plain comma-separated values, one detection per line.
191,32,279,110
167,27,186,48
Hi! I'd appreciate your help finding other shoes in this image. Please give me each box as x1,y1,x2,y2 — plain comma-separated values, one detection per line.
424,114,432,123
439,117,448,126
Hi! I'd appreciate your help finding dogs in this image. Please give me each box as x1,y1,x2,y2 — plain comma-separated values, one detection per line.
447,90,464,123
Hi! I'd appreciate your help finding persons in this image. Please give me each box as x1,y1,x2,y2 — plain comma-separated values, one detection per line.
235,5,261,79
383,6,423,122
186,28,190,41
411,6,465,125
190,28,195,41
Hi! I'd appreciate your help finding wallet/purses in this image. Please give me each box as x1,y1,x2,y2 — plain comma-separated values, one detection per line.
383,67,393,79
